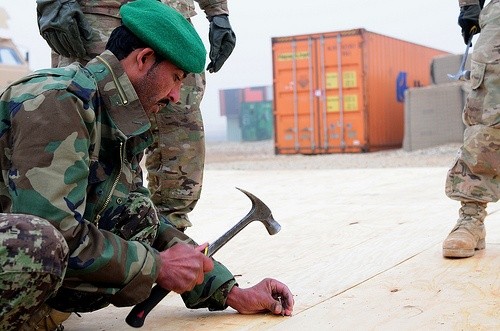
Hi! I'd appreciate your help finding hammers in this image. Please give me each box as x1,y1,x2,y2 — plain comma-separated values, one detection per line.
445,30,475,81
125,187,281,328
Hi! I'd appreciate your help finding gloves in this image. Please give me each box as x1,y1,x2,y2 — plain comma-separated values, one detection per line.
35,0,93,59
458,5,483,48
206,14,237,75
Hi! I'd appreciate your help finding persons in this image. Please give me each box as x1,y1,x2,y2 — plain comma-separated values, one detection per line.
37,0,235,233
443,0,500,259
2,0,295,331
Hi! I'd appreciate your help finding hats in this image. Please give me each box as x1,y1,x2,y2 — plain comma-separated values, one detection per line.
118,0,207,75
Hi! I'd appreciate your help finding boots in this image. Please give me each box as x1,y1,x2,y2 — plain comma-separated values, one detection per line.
20,303,76,331
441,201,488,258
